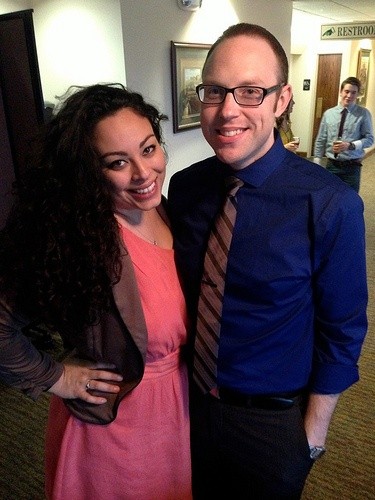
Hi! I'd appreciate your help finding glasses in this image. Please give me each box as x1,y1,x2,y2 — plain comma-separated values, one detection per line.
195,81,285,106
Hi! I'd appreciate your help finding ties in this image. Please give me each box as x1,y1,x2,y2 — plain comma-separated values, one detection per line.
334,108,348,158
190,177,246,399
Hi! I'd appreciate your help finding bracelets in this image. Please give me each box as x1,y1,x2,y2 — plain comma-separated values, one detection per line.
308,444,325,460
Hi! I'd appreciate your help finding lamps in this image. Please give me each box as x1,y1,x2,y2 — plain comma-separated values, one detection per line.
177,0,203,14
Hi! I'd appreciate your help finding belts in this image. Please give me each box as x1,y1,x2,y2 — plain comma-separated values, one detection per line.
190,389,307,410
328,158,362,169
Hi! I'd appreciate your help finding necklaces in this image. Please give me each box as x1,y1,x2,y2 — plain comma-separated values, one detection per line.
140,230,158,245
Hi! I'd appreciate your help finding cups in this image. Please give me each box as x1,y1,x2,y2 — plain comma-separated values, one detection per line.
313,156,322,165
292,137,300,148
332,137,342,150
321,157,328,167
308,156,316,163
295,151,307,159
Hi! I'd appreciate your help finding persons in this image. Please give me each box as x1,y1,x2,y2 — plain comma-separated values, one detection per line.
0,83,192,500
274,93,300,154
168,23,370,500
313,77,374,193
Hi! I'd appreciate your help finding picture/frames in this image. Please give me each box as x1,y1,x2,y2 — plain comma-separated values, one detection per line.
356,48,372,108
170,41,214,135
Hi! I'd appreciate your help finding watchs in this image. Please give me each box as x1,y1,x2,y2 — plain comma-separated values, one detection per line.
348,142,354,150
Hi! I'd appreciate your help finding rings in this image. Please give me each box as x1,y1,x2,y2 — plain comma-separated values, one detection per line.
86,382,90,388
